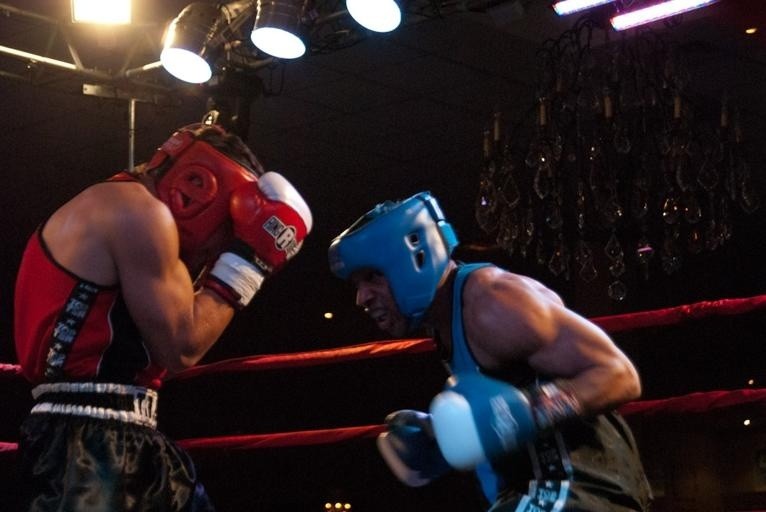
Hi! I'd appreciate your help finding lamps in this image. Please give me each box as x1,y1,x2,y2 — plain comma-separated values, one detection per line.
160,0,401,84
473,0,761,302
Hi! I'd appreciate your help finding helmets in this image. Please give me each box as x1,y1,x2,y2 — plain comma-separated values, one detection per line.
142,121,264,260
328,191,460,329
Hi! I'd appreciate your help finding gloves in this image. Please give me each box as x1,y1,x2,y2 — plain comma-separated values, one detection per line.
377,371,585,487
193,171,313,312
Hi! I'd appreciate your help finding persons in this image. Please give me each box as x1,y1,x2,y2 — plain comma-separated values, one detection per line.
15,122,313,512
328,190,653,512
